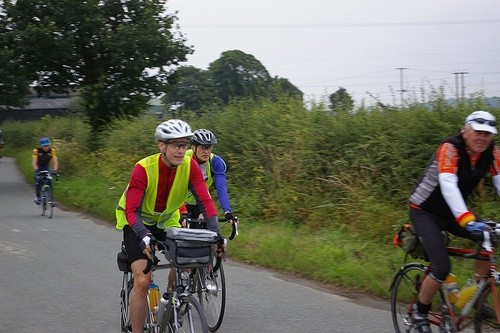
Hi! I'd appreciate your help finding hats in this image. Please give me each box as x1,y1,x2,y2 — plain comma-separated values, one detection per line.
466,111,498,134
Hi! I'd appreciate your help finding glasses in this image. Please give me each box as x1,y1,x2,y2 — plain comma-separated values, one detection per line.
464,118,498,127
162,142,188,151
196,145,212,150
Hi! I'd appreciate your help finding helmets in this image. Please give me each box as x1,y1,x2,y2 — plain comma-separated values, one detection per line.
155,119,194,142
40,138,49,146
189,129,218,146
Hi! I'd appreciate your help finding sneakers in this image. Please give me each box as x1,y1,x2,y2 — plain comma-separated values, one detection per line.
408,304,431,333
477,301,496,316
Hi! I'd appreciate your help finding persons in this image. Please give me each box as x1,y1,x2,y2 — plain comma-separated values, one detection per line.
32,137,60,208
407,110,500,327
115,119,229,333
179,128,240,294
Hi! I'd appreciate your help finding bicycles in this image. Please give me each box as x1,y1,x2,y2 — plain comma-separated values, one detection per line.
119,238,210,333
177,216,239,332
388,228,500,333
37,170,60,219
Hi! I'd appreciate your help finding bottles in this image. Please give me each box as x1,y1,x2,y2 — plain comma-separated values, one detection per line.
444,272,460,305
147,280,160,313
454,273,480,315
156,292,168,325
